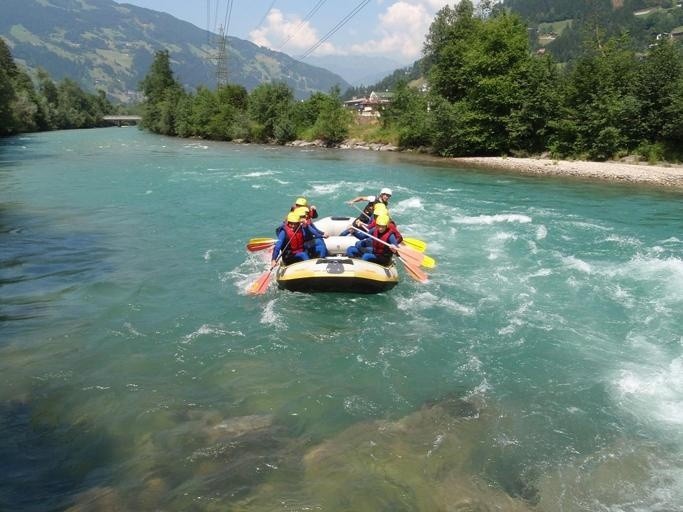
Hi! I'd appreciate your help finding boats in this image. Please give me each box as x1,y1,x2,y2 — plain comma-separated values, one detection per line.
269,214,397,295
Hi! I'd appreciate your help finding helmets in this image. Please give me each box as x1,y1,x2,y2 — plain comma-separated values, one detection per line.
375,214,389,228
373,207,388,216
295,197,307,207
293,208,306,216
299,206,310,213
287,212,301,225
379,187,392,197
373,202,385,210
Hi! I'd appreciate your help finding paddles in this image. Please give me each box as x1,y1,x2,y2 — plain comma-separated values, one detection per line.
246,238,280,251
352,204,436,283
247,223,303,295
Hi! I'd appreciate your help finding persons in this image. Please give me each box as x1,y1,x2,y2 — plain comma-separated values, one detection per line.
268,187,402,267
